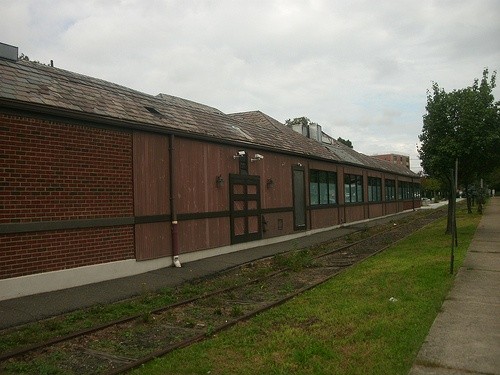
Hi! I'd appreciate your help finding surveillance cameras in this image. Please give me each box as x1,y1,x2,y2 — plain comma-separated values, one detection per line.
236,151,246,155
255,154,263,160
